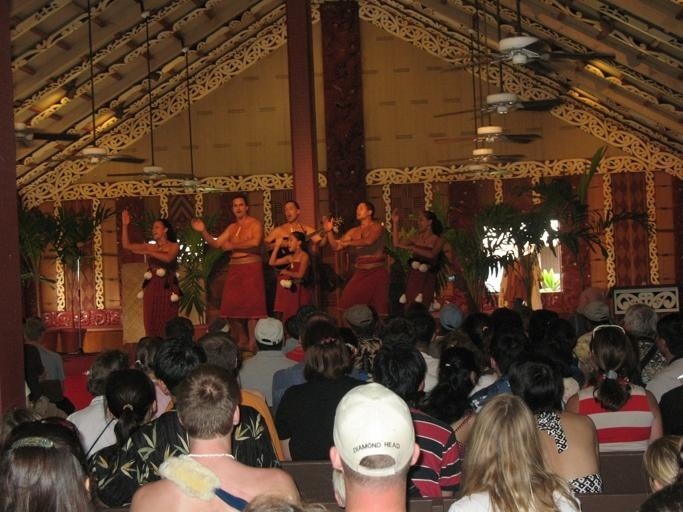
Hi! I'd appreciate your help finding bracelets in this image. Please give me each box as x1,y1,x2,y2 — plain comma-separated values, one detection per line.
325,229,332,233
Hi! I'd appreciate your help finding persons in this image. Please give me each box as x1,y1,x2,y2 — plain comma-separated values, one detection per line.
269,231,312,323
391,208,444,311
265,200,328,256
191,196,268,359
121,209,180,338
322,201,393,327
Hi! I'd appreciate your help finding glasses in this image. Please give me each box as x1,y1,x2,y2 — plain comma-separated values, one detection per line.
593,324,626,340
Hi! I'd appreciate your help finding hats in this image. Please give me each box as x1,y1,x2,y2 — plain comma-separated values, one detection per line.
254,317,283,346
332,381,414,478
583,302,609,322
440,303,464,332
343,304,374,327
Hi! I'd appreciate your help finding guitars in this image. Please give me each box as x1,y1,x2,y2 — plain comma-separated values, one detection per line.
268,216,344,271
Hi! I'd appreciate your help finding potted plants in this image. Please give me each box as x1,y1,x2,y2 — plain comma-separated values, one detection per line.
15,201,68,355
44,196,104,356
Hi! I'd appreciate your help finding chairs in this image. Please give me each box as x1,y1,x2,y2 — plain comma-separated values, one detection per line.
279,460,338,505
410,494,457,512
571,490,660,512
592,449,657,492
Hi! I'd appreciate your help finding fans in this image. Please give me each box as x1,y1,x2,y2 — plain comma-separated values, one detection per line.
113,36,191,185
435,0,615,171
14,112,81,144
39,0,145,166
160,42,227,197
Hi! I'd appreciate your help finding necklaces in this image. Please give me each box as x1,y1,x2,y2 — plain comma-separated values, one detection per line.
288,222,304,235
155,242,168,251
